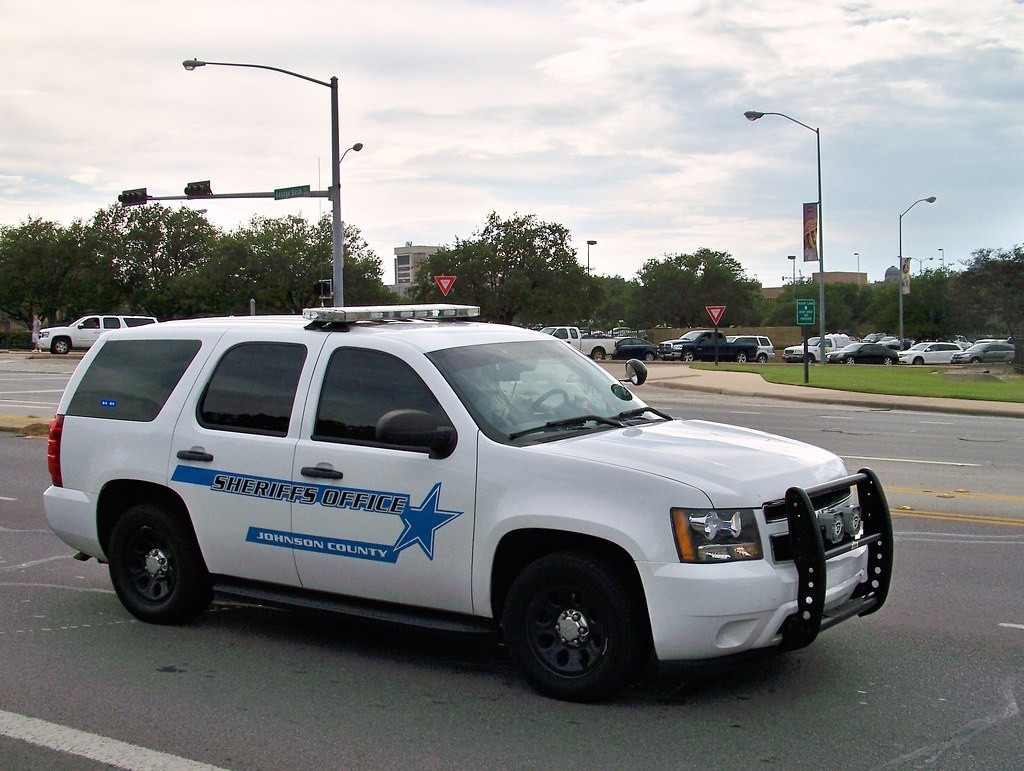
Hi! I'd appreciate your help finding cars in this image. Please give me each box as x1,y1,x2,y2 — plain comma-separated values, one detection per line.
580,327,659,361
848,332,1015,364
825,343,899,366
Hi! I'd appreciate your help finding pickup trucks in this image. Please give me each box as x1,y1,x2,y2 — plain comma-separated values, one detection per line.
539,327,617,360
659,330,758,364
782,337,844,364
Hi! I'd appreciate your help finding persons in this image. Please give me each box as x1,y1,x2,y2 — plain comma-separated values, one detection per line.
704,335,709,340
32,316,41,352
93,320,99,328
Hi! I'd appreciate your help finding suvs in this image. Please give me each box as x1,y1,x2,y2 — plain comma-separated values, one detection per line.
42,304,894,705
38,315,159,354
726,334,776,363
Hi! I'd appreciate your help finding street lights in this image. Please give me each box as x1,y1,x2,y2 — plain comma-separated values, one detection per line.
744,112,824,360
908,257,933,277
938,249,944,272
587,241,596,276
854,253,859,284
899,196,936,351
184,58,364,309
787,256,796,301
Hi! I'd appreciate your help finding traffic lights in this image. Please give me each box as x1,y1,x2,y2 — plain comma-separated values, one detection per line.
118,187,147,207
314,279,332,299
184,180,210,196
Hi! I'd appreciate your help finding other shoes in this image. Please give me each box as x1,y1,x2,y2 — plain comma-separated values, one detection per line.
32,350,38,352
39,350,41,352
